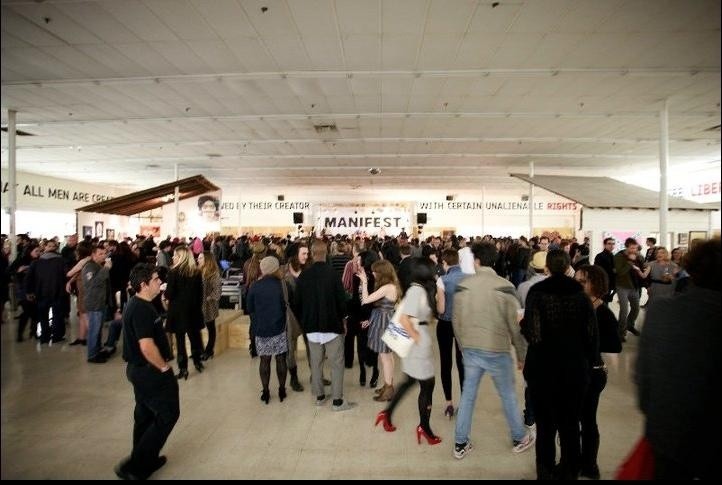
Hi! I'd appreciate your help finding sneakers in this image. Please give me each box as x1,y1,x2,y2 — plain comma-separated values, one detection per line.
452,439,474,459
511,431,536,452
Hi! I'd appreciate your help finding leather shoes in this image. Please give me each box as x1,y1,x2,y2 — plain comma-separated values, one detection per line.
87,345,117,363
290,380,304,391
144,454,167,478
69,339,88,346
309,377,359,412
112,454,145,480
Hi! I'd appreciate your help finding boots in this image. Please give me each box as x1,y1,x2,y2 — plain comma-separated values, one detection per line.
373,384,394,401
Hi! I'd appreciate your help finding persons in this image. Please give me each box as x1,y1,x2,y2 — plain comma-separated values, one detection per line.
197,195,220,223
447,232,722,480
114,263,181,480
211,230,467,444
0,228,219,378
177,211,188,234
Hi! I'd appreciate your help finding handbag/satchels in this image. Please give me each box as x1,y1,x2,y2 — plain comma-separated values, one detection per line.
282,279,302,368
380,285,427,358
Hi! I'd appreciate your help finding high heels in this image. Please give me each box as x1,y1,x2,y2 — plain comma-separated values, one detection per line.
375,410,396,431
279,388,286,402
261,389,269,404
174,349,214,380
444,405,454,420
416,424,441,445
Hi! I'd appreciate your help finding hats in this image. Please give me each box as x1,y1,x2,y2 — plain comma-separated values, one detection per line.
260,255,280,274
528,251,547,269
